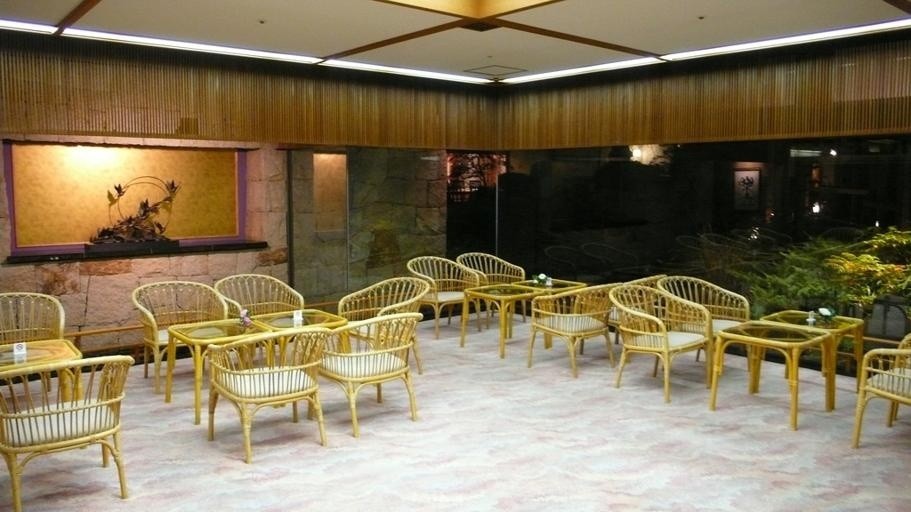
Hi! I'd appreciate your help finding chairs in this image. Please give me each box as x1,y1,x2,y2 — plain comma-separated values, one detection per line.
208,324,334,464
527,280,626,379
337,273,431,375
0,352,137,512
0,290,67,392
406,249,528,340
608,275,751,405
317,311,425,440
851,332,911,448
130,278,230,404
215,272,305,365
542,225,910,322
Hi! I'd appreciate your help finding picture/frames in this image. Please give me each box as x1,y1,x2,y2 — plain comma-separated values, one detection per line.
730,166,764,212
3,139,249,259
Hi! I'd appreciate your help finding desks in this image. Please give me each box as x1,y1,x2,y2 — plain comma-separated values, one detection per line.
459,277,588,359
709,310,867,432
163,308,347,426
0,338,88,450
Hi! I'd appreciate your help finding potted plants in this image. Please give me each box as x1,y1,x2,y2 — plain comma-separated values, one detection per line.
82,175,182,254
